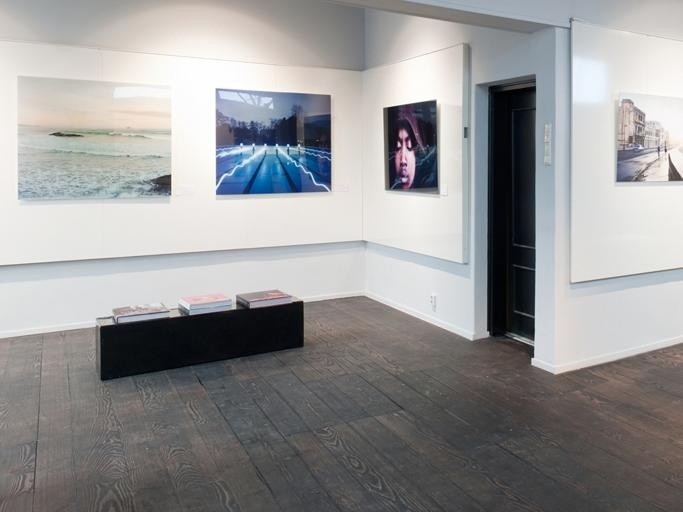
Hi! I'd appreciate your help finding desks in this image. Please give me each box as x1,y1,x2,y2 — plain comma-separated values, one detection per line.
95,292,306,384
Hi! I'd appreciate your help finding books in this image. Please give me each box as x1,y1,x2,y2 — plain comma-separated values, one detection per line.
111,301,169,325
236,288,294,309
176,304,233,315
176,291,233,309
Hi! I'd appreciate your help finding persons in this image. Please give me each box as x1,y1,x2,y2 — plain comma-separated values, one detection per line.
392,105,425,190
